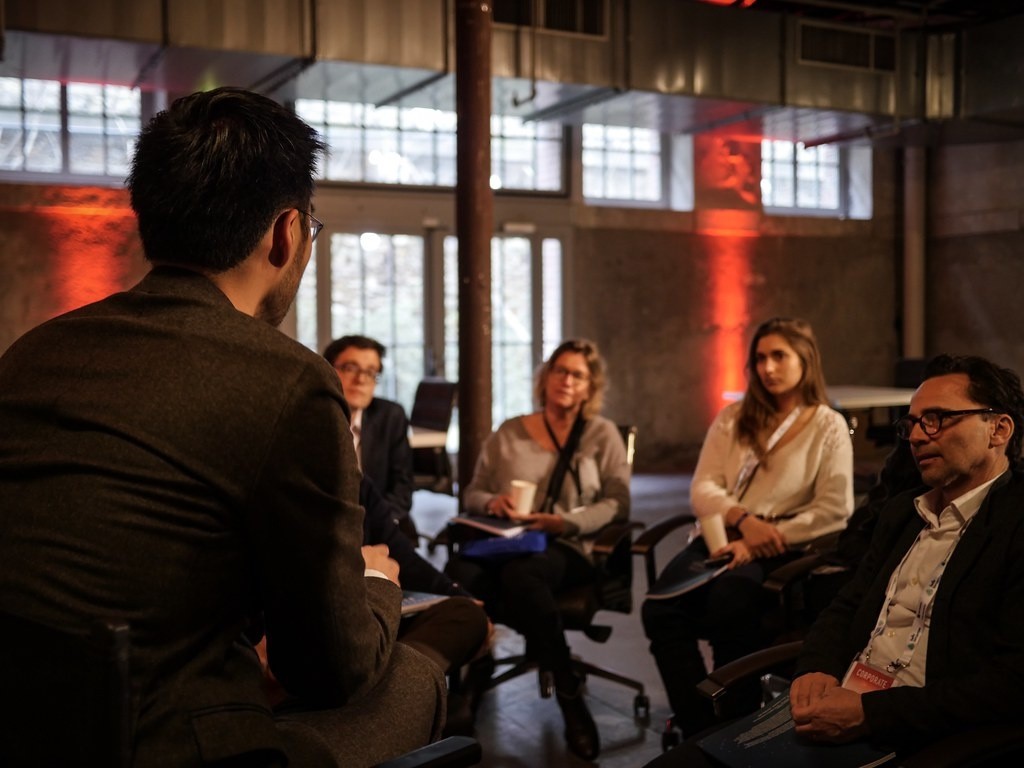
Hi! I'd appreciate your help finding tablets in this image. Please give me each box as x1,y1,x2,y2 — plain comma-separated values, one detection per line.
399,590,452,615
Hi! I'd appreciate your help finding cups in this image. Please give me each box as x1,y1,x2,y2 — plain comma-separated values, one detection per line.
698,513,727,554
511,479,538,517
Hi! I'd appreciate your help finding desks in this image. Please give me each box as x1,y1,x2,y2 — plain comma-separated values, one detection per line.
407,423,448,448
725,383,920,495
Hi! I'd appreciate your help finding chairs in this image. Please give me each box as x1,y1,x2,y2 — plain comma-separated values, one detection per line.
410,380,462,554
866,358,927,446
0,606,485,768
448,423,651,723
629,475,1024,768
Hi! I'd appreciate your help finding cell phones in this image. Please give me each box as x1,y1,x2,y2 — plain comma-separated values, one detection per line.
704,555,732,568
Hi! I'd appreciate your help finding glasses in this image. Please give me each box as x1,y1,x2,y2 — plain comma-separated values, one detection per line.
277,208,324,243
892,409,1020,440
549,364,590,386
332,361,382,386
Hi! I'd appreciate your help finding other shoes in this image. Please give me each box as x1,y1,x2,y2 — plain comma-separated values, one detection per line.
456,649,495,703
556,687,601,762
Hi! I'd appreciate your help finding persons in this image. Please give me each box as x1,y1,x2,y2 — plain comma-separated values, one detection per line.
445,339,632,760
641,319,1024,768
0,86,487,768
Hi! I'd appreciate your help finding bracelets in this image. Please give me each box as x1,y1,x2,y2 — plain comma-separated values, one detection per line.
736,512,755,529
